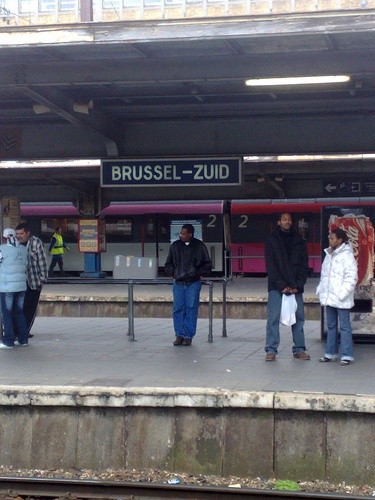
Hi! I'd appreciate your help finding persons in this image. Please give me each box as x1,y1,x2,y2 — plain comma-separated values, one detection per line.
264,211,311,360
48,227,71,275
315,227,359,366
0,228,29,349
15,223,48,340
164,224,213,345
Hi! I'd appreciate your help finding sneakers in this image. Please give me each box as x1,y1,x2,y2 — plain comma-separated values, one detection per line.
173,337,191,345
295,351,310,360
0,341,14,349
13,340,29,346
320,355,336,362
341,361,351,365
266,351,277,361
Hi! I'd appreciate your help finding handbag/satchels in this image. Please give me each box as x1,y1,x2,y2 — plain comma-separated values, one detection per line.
279,293,297,326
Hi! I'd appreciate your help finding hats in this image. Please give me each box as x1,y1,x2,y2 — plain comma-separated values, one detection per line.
3,228,16,238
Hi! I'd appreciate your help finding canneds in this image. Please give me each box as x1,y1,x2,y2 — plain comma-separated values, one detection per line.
168,479,180,485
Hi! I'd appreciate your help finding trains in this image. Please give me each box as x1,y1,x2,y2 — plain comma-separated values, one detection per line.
18,194,375,277
